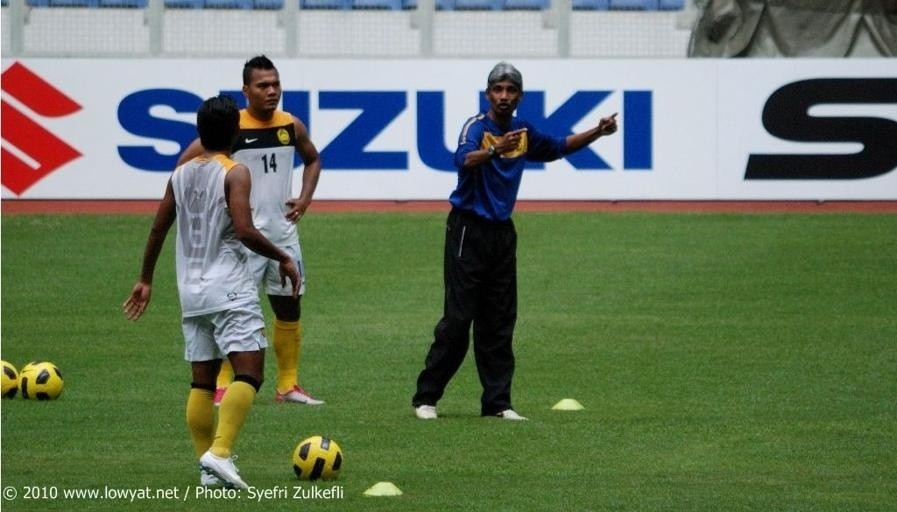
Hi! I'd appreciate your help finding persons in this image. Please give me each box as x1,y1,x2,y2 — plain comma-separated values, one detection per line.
122,94,302,492
410,61,620,422
177,55,326,407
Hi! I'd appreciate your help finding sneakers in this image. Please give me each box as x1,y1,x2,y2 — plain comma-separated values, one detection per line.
199,449,252,490
415,404,439,421
274,382,327,406
213,386,226,406
495,408,530,421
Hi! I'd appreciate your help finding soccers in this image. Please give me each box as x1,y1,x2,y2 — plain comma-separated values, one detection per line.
1,359,18,400
292,436,343,480
18,361,64,401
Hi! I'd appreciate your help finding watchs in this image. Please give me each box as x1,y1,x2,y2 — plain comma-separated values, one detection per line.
487,144,496,158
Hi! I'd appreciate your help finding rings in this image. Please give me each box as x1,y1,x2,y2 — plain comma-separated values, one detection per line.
294,211,300,216
